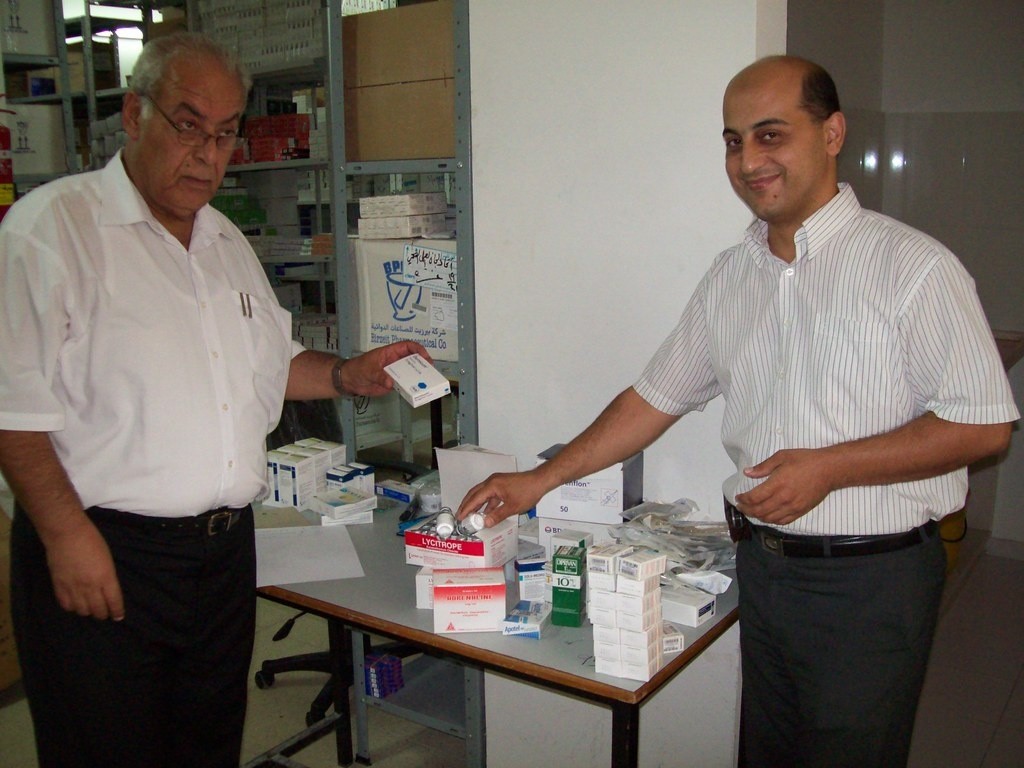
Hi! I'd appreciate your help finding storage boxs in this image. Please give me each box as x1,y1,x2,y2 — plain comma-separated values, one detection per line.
333,464,361,490
433,567,506,634
261,438,353,512
551,607,587,628
0,507,22,691
320,509,373,527
347,462,375,495
537,517,620,562
415,567,432,610
536,443,644,523
549,529,593,568
504,538,546,583
307,485,378,520
551,567,586,613
375,478,418,504
311,390,403,450
552,546,587,575
0,0,458,362
503,600,552,640
404,443,519,569
587,542,716,683
383,353,452,409
512,559,548,601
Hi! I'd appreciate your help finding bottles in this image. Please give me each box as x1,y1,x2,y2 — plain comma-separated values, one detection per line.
436,506,455,535
455,514,484,536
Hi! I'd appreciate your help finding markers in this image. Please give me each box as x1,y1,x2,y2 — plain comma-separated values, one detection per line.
399,499,421,523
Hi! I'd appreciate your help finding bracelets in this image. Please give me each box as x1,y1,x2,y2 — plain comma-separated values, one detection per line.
331,357,361,402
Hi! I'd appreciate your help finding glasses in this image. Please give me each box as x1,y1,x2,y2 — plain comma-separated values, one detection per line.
141,93,248,150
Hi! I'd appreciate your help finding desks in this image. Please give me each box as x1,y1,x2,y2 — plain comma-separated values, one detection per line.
241,494,740,768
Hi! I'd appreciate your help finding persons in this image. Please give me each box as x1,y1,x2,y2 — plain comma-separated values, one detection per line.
1,34,434,768
453,54,1022,768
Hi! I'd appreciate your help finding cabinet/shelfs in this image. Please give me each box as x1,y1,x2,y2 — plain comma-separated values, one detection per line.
319,0,485,768
64,14,145,103
0,0,79,184
84,0,391,279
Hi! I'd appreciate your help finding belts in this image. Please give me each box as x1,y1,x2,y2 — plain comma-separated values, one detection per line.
84,506,238,537
724,499,937,555
